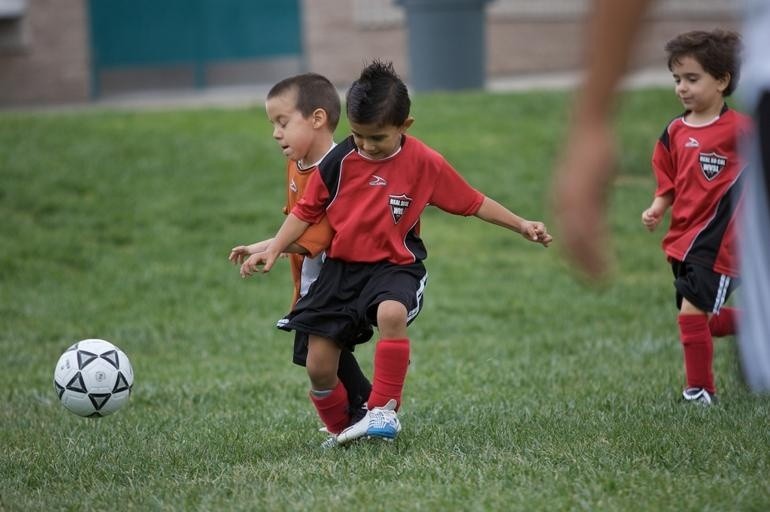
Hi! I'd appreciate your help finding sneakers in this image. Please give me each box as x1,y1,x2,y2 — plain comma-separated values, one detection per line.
339,390,374,448
313,425,344,451
366,397,403,441
680,385,716,408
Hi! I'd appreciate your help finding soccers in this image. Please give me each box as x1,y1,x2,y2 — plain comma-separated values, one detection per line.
53,339,133,418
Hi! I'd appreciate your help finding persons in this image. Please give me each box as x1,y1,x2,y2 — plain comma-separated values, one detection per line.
239,60,556,452
638,26,761,409
227,71,371,425
